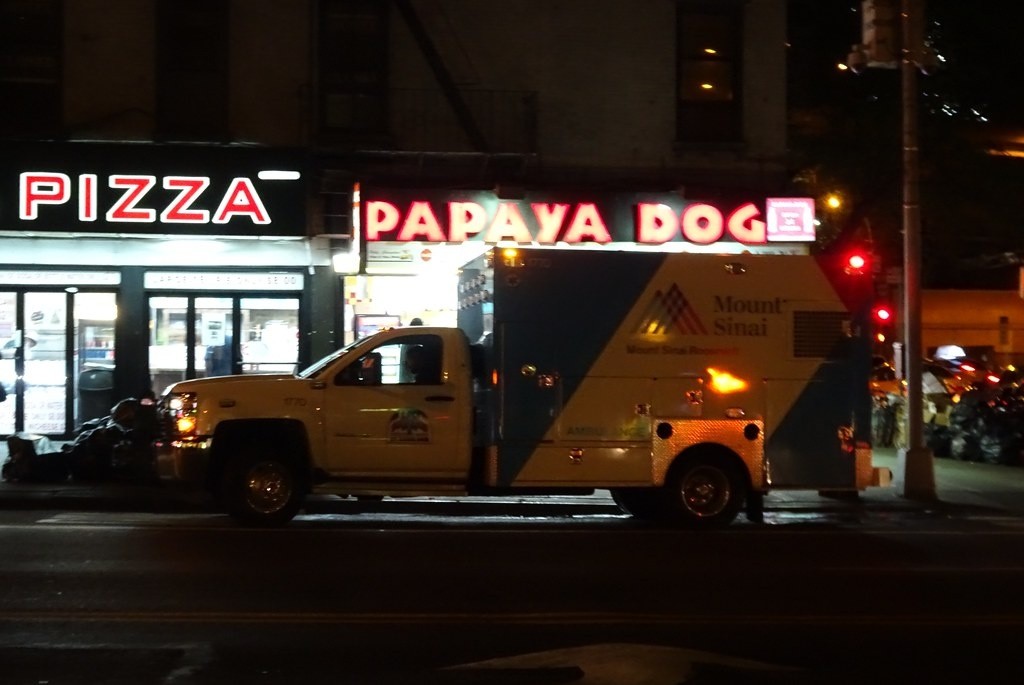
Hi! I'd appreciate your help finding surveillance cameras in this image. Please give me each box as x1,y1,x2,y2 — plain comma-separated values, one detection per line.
847,52,868,75
920,56,940,76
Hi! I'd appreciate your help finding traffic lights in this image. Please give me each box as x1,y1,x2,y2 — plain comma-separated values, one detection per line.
844,247,869,297
871,303,895,347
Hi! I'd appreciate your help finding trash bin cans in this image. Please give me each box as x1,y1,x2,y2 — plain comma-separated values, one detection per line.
79,368,115,424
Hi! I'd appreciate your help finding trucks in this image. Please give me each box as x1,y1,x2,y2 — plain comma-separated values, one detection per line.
152,235,894,533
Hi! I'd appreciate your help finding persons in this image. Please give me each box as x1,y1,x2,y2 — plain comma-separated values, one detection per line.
406,346,442,384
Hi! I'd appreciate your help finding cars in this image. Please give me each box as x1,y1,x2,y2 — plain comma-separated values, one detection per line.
869,354,1001,435
